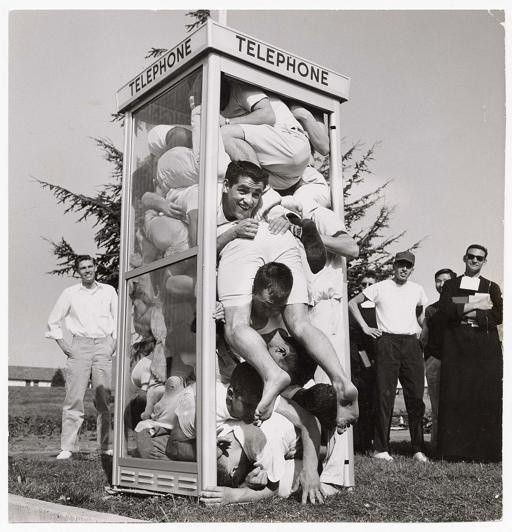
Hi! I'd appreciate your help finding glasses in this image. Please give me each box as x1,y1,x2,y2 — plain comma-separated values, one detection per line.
467,253,485,262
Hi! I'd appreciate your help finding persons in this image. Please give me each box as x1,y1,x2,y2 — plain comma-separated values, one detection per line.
131,71,360,505
350,245,503,463
46,254,118,459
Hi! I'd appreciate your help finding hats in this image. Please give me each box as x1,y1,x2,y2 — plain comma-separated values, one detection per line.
396,252,415,265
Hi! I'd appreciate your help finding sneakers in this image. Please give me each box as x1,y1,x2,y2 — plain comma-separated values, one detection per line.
413,452,430,464
373,450,393,461
100,449,113,456
56,451,74,460
